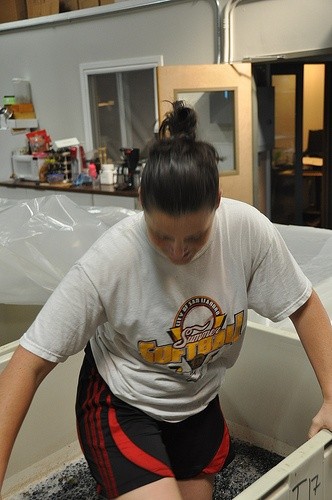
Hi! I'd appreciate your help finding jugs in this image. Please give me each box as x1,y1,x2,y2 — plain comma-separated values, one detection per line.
12,78,32,104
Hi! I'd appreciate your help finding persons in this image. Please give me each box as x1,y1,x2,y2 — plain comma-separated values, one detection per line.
0,100,332,500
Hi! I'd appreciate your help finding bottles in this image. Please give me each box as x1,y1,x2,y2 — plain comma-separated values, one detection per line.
70,147,79,181
99,164,118,185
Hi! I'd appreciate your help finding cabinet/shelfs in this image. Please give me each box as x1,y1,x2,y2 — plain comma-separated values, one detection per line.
0,180,138,209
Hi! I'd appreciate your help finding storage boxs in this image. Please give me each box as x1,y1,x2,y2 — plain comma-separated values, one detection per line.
0,308,331,499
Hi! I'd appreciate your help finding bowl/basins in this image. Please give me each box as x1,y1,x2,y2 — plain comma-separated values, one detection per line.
47,174,64,184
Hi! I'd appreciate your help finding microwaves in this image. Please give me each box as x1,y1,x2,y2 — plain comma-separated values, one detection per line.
11,154,48,181
